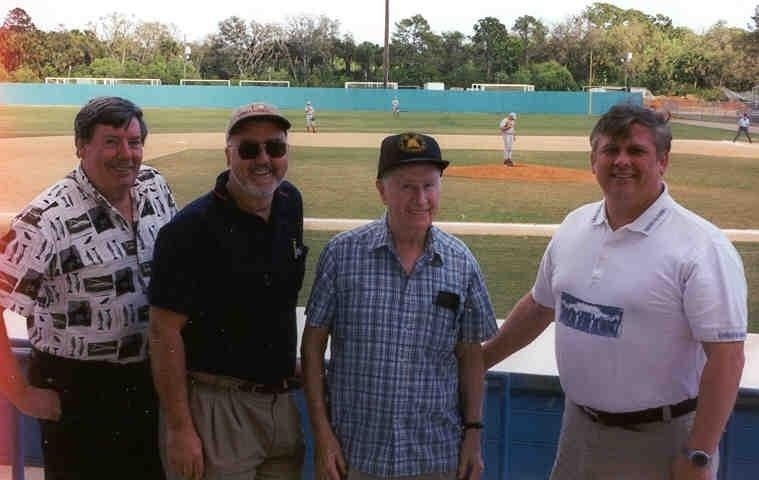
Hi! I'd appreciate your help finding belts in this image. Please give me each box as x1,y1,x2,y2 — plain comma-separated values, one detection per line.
582,398,697,425
198,376,292,395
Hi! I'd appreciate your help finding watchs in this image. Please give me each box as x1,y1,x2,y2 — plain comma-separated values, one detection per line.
681,445,717,468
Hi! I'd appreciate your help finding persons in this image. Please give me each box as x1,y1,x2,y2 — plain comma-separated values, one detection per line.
475,101,749,480
138,99,311,479
1,94,184,478
391,94,401,118
733,110,755,147
294,131,502,479
303,100,314,132
496,112,516,169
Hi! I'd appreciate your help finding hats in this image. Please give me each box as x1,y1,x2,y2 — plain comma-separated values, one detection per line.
378,133,450,178
225,103,290,139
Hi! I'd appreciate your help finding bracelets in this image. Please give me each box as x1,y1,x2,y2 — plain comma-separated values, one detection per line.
459,422,485,431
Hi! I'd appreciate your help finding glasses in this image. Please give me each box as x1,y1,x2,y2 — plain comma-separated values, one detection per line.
229,140,288,160
